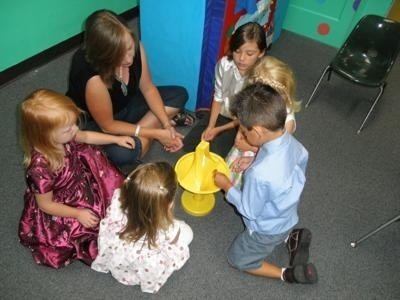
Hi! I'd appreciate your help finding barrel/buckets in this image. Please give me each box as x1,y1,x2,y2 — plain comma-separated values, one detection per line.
173,111,195,127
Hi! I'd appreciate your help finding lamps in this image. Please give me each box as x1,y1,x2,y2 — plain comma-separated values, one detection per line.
304,14,400,134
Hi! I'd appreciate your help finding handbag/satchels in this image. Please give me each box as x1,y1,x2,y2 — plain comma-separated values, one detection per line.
135,125,141,137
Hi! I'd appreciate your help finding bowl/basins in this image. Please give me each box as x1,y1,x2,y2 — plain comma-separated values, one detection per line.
113,66,128,97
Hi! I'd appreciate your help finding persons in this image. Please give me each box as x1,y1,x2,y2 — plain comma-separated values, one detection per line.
18,89,126,270
224,55,304,192
91,162,194,294
66,9,194,166
183,22,267,158
213,83,318,284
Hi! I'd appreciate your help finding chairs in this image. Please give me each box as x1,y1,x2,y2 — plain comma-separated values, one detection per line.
284,263,318,284
287,228,311,266
